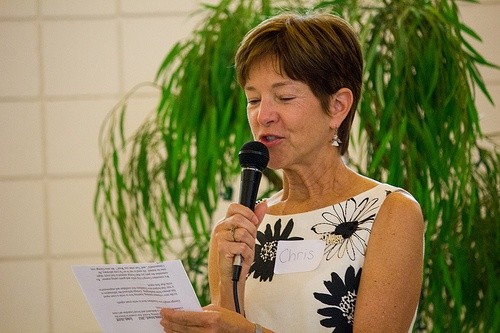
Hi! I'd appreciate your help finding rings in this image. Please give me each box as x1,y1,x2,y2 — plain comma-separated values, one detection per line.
230,225,240,242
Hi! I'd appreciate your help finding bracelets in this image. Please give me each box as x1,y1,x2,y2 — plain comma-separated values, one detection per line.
254,323,262,333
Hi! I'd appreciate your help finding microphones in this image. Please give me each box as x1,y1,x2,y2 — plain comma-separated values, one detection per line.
232,141,269,281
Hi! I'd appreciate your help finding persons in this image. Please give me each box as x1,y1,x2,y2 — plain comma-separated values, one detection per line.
160,14,425,333
